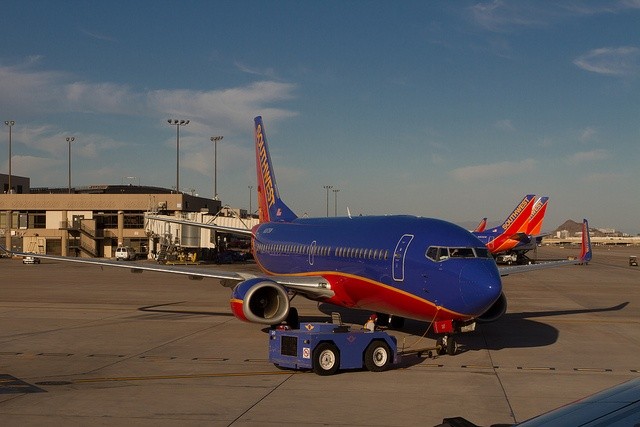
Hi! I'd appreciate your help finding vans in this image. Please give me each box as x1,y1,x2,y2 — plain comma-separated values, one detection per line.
115,246,137,260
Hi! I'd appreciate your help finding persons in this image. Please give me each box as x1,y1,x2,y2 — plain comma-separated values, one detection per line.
363,314,378,331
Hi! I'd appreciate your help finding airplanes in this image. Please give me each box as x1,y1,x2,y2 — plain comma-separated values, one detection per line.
0,116,593,355
465,194,553,265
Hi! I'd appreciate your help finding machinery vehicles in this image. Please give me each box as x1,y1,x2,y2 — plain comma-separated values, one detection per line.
268,312,401,376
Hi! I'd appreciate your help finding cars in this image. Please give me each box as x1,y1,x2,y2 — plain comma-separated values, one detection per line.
629,255,638,266
21,251,41,265
